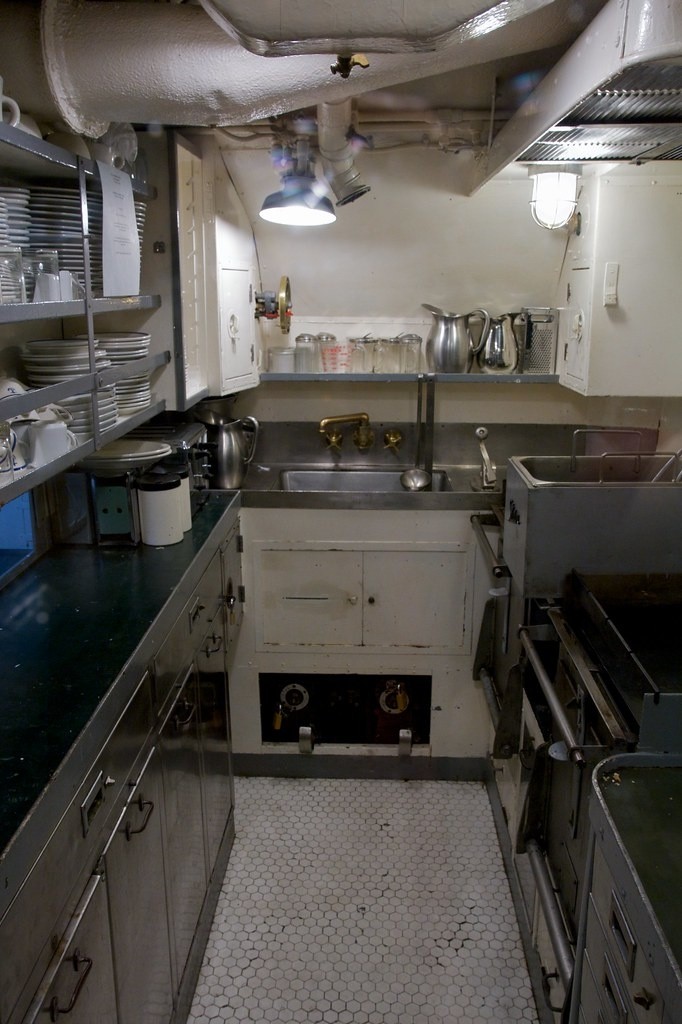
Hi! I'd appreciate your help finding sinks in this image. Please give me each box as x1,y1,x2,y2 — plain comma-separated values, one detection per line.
277,466,457,494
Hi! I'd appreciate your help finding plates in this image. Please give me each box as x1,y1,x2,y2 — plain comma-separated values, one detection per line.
1,184,171,467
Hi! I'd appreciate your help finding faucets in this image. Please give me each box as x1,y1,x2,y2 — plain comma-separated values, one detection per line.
318,412,375,449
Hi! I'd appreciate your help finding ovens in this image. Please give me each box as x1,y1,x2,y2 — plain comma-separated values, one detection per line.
496,610,633,913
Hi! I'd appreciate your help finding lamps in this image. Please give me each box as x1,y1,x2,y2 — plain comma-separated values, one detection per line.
527,165,583,230
259,165,338,226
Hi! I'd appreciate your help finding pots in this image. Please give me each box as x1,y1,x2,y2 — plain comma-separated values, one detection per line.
196,393,237,417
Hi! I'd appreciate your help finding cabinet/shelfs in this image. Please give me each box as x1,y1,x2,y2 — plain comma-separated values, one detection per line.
0,492,248,1024
134,131,260,411
0,122,172,511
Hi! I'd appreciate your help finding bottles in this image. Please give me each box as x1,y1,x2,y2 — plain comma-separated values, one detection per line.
294,333,318,374
378,338,400,373
400,333,422,374
316,332,337,373
347,338,377,373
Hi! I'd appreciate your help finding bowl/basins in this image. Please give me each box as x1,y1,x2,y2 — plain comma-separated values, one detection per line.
48,131,91,160
3,111,42,139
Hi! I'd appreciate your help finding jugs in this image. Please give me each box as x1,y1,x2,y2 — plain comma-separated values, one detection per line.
193,409,259,488
471,313,520,374
421,304,490,374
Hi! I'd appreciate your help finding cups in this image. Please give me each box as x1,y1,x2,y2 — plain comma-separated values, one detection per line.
70,273,79,300
36,249,60,301
0,246,26,304
268,349,295,373
0,75,20,128
59,270,71,301
86,142,125,170
0,373,77,489
